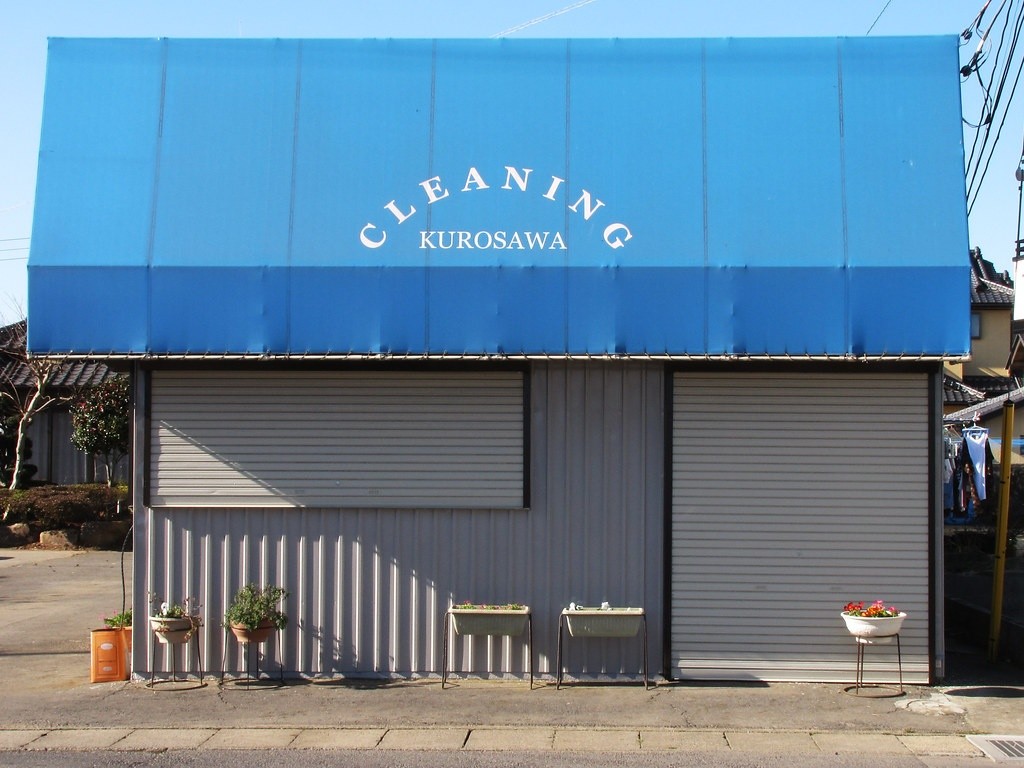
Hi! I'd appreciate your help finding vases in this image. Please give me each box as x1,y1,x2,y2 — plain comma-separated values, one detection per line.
447,604,532,638
112,626,132,653
562,607,645,639
840,610,908,644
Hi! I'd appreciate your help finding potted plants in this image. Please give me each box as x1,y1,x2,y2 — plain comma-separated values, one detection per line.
218,582,291,643
69,372,134,551
145,590,204,643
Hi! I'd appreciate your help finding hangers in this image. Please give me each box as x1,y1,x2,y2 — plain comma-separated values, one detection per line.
944,419,990,445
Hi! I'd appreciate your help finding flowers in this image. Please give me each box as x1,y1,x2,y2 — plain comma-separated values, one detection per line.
844,600,901,618
98,608,132,629
455,600,524,610
